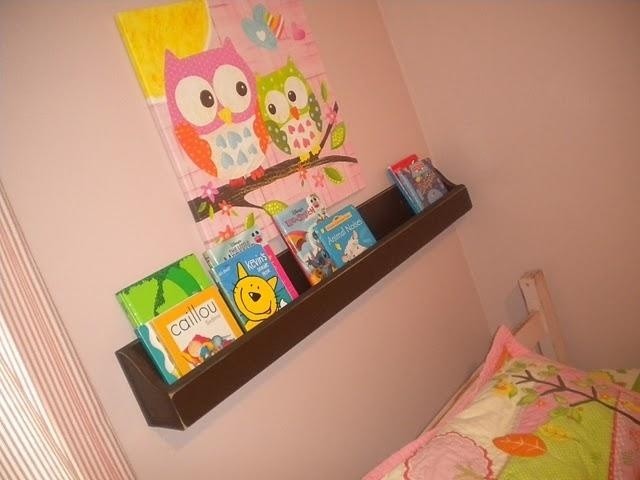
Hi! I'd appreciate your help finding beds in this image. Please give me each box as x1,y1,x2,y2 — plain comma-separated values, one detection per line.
339,267,640,480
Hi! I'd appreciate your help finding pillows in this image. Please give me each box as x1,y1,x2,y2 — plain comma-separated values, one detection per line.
359,324,640,480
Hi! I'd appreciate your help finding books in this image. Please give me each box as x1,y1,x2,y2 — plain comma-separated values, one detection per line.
386,154,447,213
117,223,299,386
273,194,376,287
